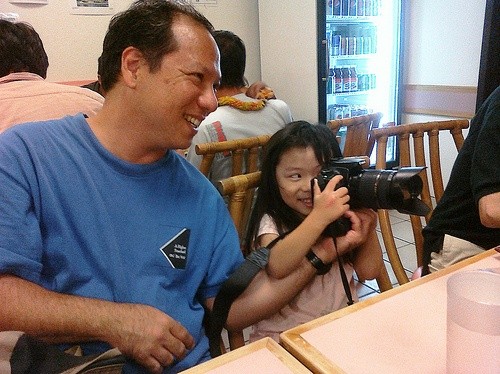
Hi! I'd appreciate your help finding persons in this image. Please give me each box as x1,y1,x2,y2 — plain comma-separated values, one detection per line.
177,30,293,238
422,86,500,277
0,0,377,374
0,19,107,134
253,120,383,344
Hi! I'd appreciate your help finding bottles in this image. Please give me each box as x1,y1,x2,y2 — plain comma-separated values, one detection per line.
325,0,378,17
328,103,371,128
326,26,375,56
328,65,376,93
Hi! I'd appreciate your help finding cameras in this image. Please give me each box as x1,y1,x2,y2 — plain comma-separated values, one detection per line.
311,155,432,239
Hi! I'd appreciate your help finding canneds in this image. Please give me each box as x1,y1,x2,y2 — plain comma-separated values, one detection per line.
332,35,376,55
357,73,376,91
325,0,381,16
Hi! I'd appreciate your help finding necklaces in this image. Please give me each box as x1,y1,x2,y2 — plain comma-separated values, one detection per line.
218,89,272,111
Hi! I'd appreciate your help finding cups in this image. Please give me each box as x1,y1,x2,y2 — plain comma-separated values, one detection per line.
445,271,500,374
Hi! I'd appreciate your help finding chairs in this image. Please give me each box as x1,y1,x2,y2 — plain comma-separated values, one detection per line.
195,113,469,354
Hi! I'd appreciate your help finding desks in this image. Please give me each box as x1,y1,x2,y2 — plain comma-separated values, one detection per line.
279,243,500,374
176,336,314,374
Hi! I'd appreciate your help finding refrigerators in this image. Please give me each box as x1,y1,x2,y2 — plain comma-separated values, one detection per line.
256,0,406,170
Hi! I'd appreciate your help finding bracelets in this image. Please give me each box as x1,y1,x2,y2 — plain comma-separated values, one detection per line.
305,250,332,275
